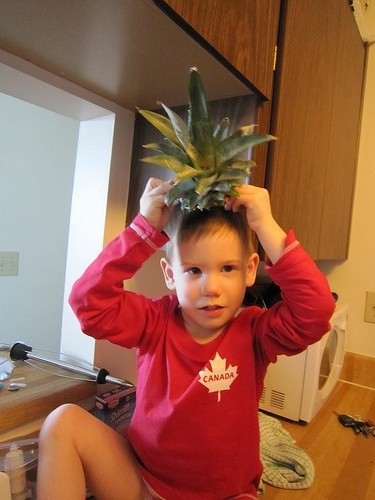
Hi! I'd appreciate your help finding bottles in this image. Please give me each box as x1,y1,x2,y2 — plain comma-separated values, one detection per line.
4,442,26,494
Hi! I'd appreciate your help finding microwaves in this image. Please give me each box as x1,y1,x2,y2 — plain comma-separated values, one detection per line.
257,306,348,424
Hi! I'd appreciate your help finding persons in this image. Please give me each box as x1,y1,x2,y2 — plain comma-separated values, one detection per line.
37,172,336,500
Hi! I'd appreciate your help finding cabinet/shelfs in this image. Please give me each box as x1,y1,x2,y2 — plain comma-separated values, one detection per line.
0,0,282,115
128,0,368,263
